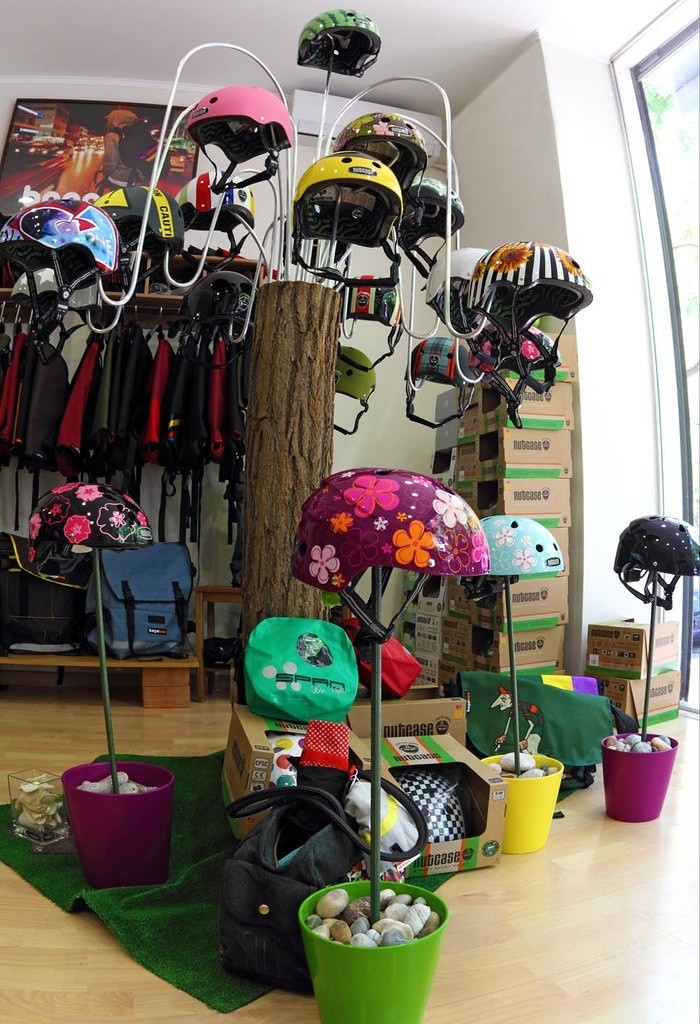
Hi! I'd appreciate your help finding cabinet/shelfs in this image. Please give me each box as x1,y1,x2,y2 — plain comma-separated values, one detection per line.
0,250,266,323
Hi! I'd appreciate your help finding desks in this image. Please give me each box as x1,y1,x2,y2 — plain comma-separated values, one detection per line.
192,584,243,705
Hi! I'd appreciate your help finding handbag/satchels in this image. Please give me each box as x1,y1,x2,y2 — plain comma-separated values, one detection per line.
338,618,422,697
243,617,358,723
218,767,429,995
456,669,639,791
346,769,421,878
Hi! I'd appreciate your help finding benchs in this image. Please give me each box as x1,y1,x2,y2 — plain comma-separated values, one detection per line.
0,651,200,709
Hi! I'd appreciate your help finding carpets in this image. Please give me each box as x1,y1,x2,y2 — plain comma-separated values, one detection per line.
0,748,581,1015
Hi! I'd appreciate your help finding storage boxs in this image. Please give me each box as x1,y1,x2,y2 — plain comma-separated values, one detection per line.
398,334,578,685
584,618,681,728
218,685,508,878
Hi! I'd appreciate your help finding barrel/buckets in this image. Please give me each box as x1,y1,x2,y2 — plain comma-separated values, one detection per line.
61,761,175,889
481,754,564,853
601,734,679,822
299,880,449,1023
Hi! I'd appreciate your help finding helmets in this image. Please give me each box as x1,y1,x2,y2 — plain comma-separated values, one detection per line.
29,481,153,576
0,8,593,435
614,514,700,582
478,515,564,575
289,466,491,594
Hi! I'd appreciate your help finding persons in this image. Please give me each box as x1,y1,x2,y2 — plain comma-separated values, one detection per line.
97,109,144,198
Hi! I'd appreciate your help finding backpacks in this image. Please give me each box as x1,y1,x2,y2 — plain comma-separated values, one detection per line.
86,541,197,660
0,531,87,656
